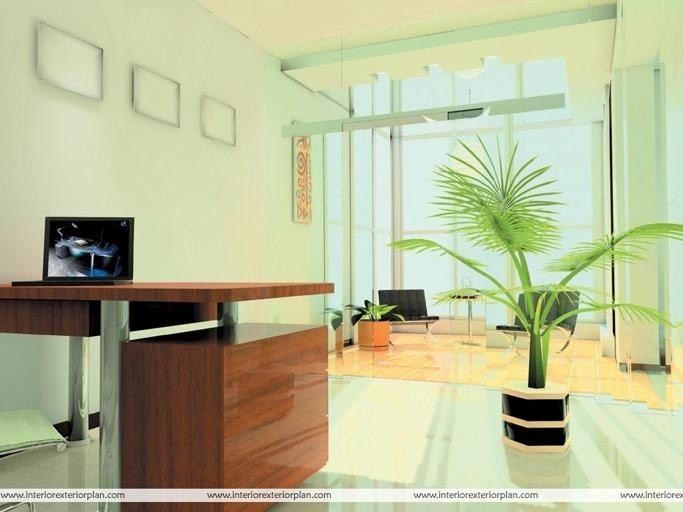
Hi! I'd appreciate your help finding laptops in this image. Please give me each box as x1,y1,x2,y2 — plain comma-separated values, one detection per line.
10,216,134,286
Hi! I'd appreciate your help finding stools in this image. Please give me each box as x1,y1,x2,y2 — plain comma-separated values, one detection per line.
0,410,68,511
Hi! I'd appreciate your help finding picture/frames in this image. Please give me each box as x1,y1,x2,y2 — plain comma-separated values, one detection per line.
291,120,312,225
199,93,237,147
36,16,105,100
130,64,181,126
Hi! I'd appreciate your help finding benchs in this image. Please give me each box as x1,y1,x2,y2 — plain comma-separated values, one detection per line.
497,291,580,360
378,289,439,341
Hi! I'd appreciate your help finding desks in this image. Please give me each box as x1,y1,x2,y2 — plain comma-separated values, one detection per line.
0,280,334,504
451,294,482,347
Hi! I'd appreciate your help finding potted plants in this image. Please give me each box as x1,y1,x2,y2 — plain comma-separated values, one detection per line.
383,136,682,455
344,300,406,352
324,307,344,353
